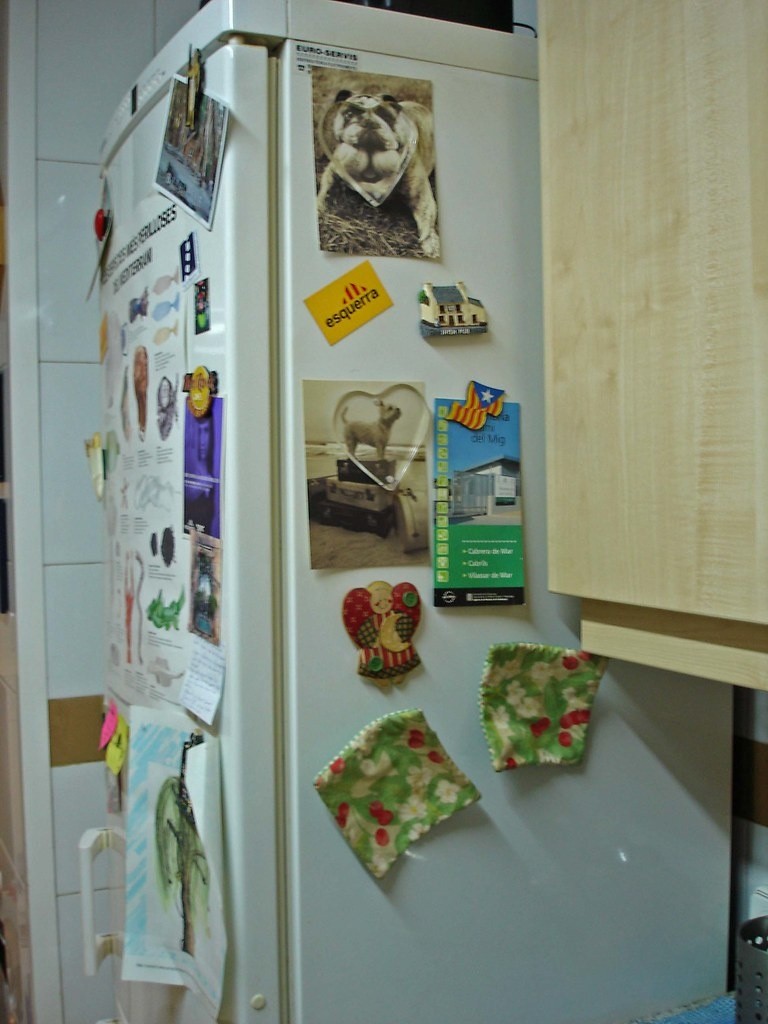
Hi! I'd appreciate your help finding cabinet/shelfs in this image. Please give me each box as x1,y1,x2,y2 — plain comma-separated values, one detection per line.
535,0,768,688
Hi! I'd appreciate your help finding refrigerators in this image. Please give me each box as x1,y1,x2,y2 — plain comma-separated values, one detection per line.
77,0,733,1024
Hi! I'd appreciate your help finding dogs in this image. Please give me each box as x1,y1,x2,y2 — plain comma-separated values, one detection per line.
317,87,441,263
334,397,404,466
